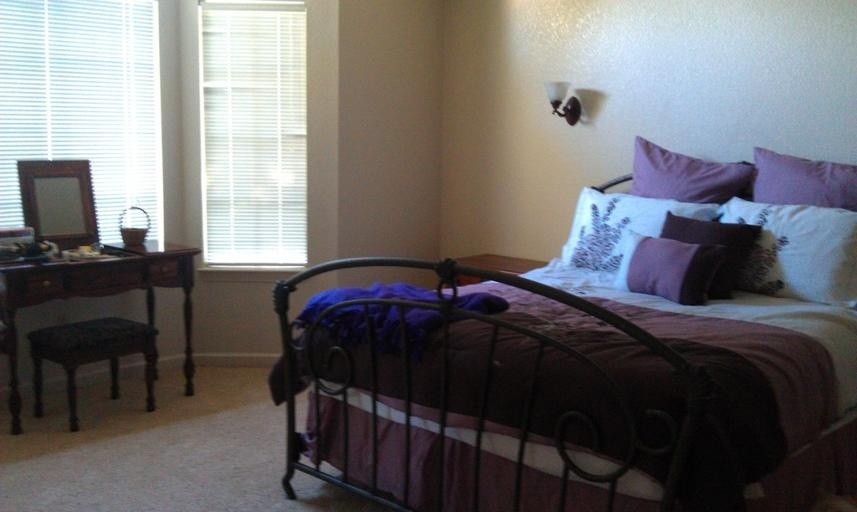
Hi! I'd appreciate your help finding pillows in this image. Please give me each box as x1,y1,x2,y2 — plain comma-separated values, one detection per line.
629,236,716,309
660,211,762,303
752,146,856,212
721,196,856,310
630,136,757,204
545,186,719,291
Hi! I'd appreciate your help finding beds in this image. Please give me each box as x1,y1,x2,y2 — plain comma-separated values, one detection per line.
270,161,857,512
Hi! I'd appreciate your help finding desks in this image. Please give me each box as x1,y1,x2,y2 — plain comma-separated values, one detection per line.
0,238,203,433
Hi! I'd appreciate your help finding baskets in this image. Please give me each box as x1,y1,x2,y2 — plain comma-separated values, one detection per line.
119,206,151,246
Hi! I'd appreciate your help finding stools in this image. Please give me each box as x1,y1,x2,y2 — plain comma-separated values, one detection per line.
25,315,161,431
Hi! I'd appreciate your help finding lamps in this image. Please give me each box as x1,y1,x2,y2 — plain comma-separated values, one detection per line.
541,80,583,126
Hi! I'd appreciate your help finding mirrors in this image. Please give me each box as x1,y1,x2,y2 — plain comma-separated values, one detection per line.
16,157,100,250
